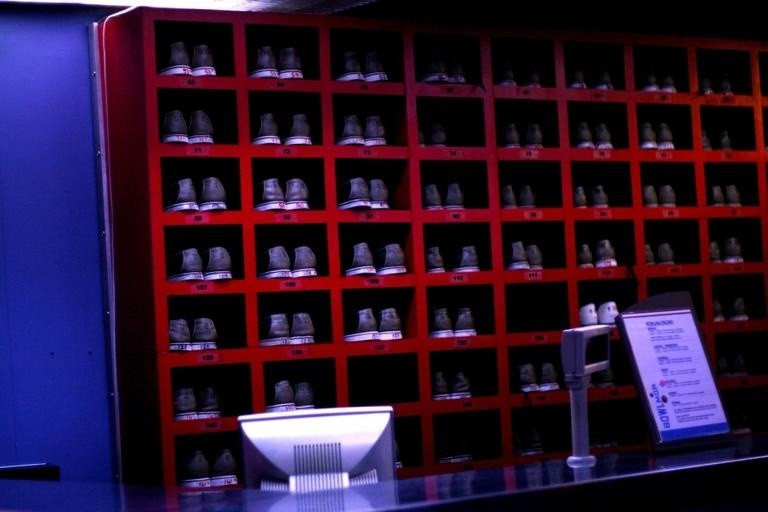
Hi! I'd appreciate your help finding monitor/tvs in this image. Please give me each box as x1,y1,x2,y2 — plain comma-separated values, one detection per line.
238,405,395,493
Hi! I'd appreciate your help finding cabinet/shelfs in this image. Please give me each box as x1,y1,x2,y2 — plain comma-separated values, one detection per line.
85,0,768,488
